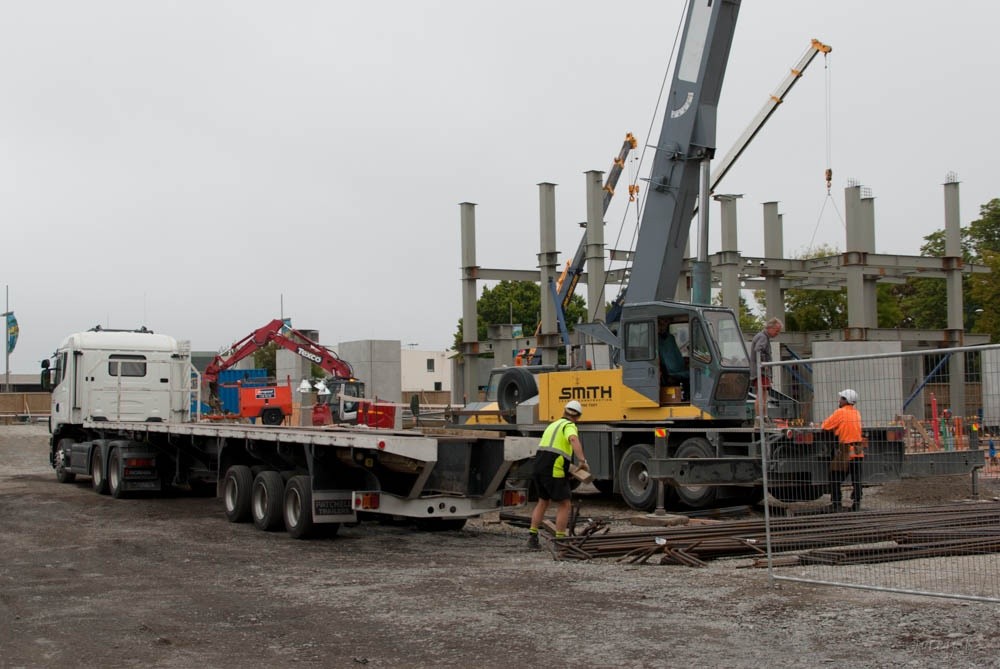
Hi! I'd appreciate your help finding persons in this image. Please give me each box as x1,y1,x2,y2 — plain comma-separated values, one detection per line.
749,319,783,428
821,389,864,511
526,400,590,549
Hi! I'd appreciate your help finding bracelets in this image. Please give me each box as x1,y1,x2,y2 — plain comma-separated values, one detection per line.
580,460,586,463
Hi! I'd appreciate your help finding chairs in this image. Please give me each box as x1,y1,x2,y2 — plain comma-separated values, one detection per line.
661,333,692,382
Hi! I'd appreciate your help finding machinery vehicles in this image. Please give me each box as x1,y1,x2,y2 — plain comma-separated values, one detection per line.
201,318,356,432
421,0,987,526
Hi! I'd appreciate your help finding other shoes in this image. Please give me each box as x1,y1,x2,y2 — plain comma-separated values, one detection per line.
848,503,860,512
526,532,539,547
754,418,772,429
823,503,843,513
554,540,568,550
764,417,776,427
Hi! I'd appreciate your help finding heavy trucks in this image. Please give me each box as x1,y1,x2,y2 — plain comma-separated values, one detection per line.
312,373,446,439
40,323,543,541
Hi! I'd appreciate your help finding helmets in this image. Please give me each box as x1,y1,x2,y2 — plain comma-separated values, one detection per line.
566,401,582,415
839,389,858,404
